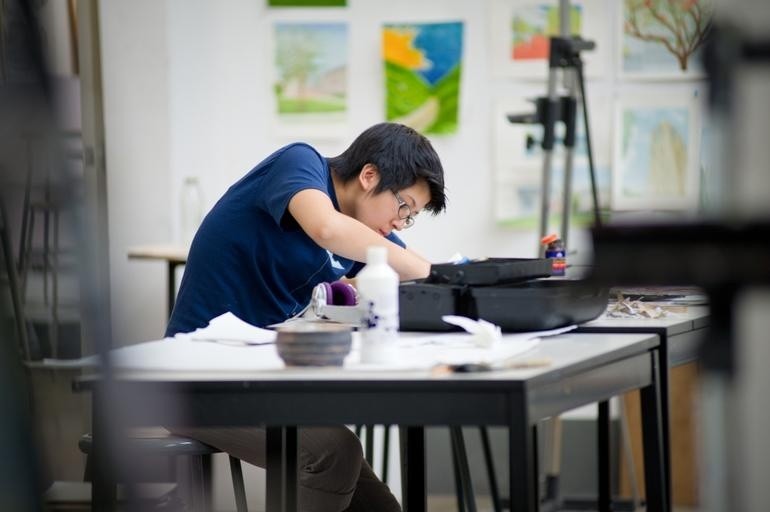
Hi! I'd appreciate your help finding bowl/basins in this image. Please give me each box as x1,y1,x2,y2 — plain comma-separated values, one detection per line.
275,324,353,370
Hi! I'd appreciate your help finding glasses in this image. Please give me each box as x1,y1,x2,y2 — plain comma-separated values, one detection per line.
391,189,415,229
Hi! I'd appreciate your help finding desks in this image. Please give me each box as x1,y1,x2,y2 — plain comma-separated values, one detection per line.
380,285,710,510
71,332,662,512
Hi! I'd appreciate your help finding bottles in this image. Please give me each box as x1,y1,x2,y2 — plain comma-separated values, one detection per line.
543,235,565,276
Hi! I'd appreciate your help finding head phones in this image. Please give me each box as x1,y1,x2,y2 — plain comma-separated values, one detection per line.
312,280,369,324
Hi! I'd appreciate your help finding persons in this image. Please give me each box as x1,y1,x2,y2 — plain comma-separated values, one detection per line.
161,121,447,511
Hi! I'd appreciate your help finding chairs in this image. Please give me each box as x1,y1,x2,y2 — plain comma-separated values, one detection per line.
79,251,260,512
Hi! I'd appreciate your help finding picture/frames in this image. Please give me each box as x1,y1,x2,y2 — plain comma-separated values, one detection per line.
498,80,609,216
489,0,614,79
614,3,718,82
611,86,705,216
